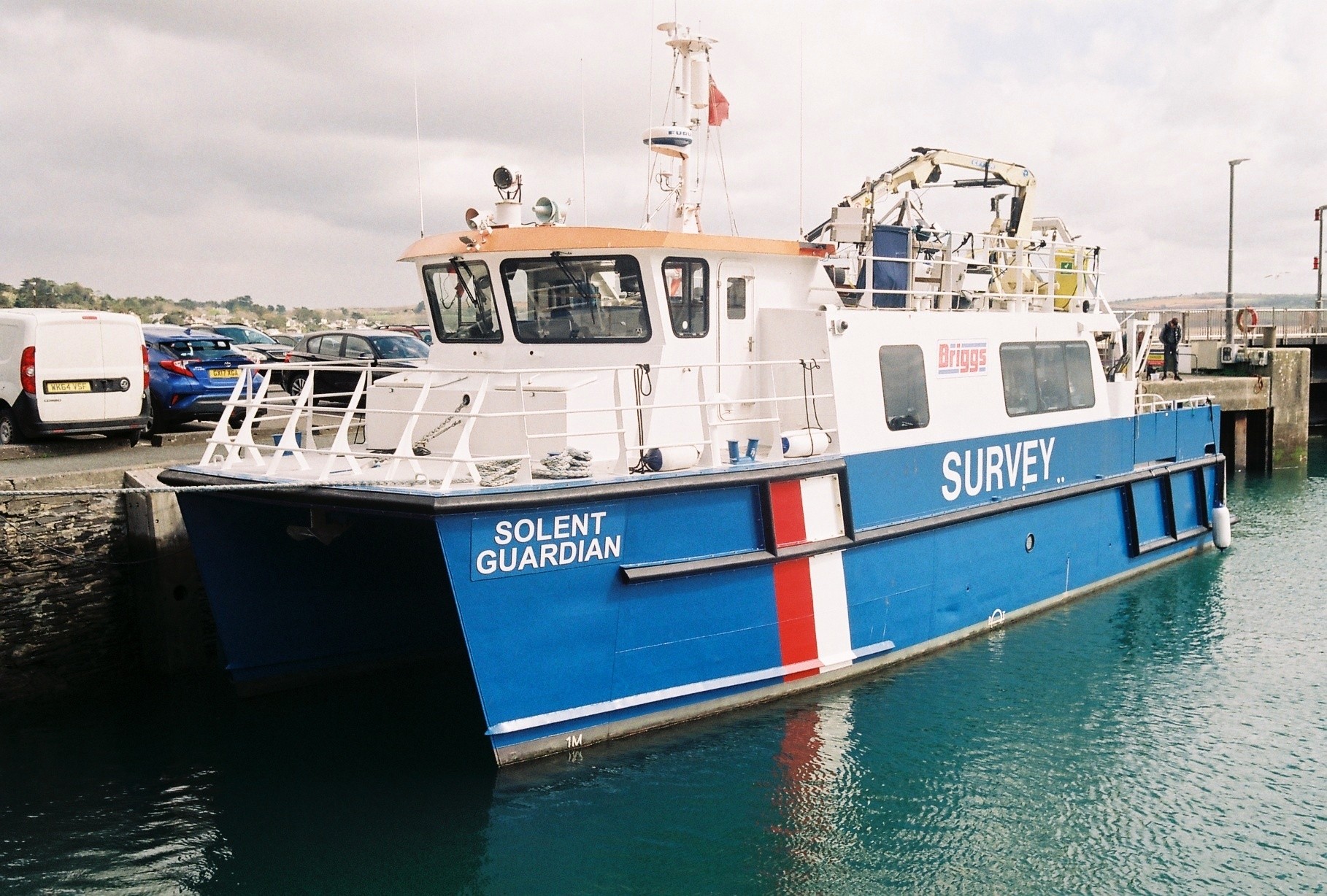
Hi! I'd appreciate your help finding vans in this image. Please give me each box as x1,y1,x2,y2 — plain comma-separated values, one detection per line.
1,306,154,445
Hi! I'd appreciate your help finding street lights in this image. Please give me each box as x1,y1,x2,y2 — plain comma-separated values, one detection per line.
1223,159,1253,343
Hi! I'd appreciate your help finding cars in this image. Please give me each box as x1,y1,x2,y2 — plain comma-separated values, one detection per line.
210,323,433,376
280,328,431,410
142,327,267,441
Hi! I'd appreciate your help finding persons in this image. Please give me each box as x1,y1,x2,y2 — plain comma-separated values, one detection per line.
1159,318,1182,381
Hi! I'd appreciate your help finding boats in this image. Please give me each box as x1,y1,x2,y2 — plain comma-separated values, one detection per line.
160,12,1232,769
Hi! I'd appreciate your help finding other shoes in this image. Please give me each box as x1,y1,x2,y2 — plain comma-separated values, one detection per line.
1161,374,1166,381
1174,375,1182,381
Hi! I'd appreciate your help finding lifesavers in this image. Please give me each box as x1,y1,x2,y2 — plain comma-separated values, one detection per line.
1236,307,1258,333
